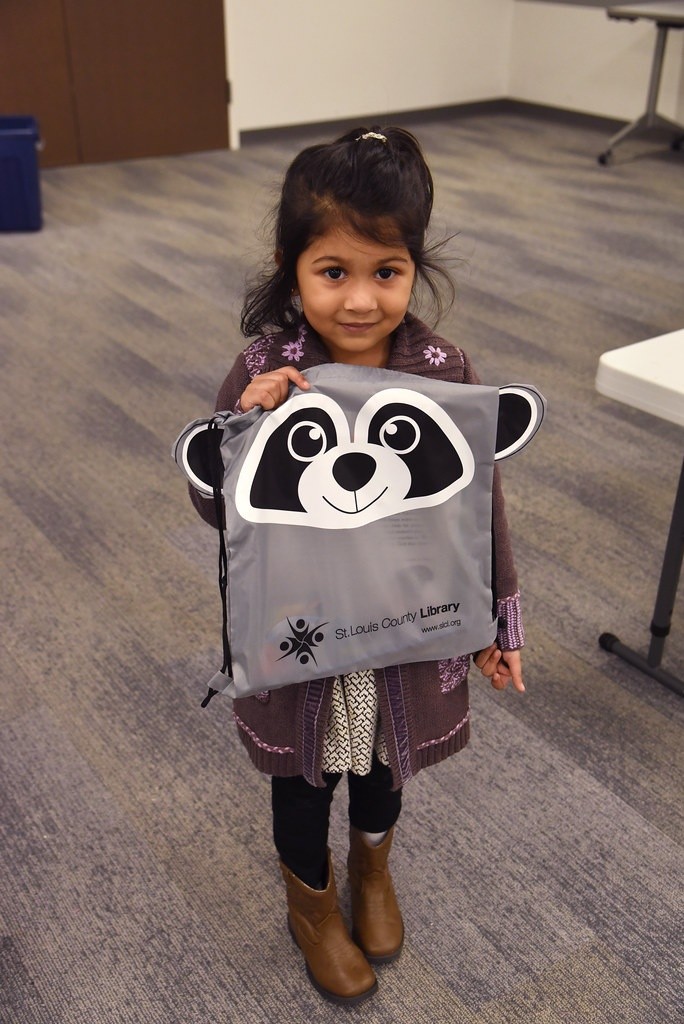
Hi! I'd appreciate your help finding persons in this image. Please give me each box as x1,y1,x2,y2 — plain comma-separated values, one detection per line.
181,120,529,1006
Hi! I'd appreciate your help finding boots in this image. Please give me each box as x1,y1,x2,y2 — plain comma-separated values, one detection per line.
276,846,380,1008
345,825,405,964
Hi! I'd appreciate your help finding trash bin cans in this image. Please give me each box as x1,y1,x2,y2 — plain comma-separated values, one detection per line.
1,115,45,232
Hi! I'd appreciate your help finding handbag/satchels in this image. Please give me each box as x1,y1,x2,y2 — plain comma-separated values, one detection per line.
199,360,502,717
